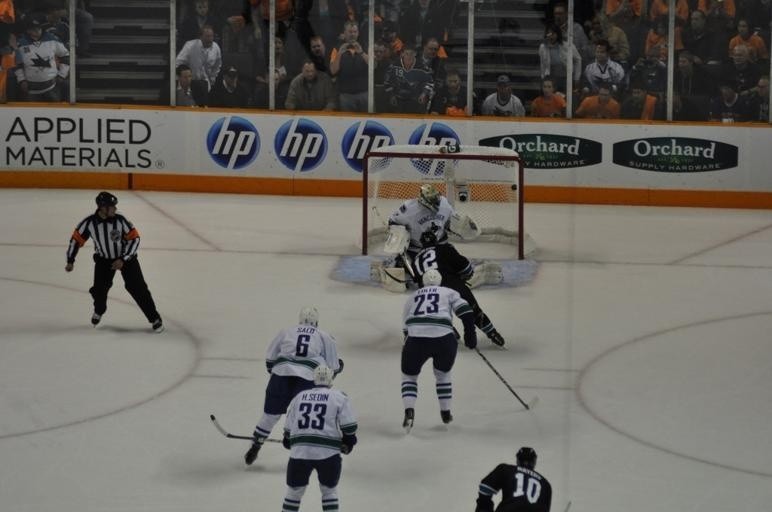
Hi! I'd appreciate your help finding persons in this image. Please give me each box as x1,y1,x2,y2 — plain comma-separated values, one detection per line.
531,0,769,123
281,363,359,512
244,306,345,472
0,0,94,100
474,445,553,512
398,269,478,435
65,190,165,330
482,74,526,116
386,182,480,292
407,231,505,350
173,0,479,116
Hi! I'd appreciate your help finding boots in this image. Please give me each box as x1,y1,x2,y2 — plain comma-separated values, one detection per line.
480,322,504,345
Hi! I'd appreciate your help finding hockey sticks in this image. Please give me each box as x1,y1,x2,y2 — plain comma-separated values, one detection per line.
209,414,282,444
472,346,539,408
565,501,572,512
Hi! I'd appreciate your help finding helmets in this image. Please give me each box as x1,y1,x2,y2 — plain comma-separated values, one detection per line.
421,220,448,246
423,269,442,286
300,305,318,322
517,447,537,461
96,192,117,206
418,184,440,213
314,365,332,385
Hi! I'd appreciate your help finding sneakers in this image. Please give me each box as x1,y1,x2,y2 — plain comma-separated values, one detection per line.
403,409,414,426
153,317,162,329
441,410,452,423
245,445,260,464
92,311,101,324
